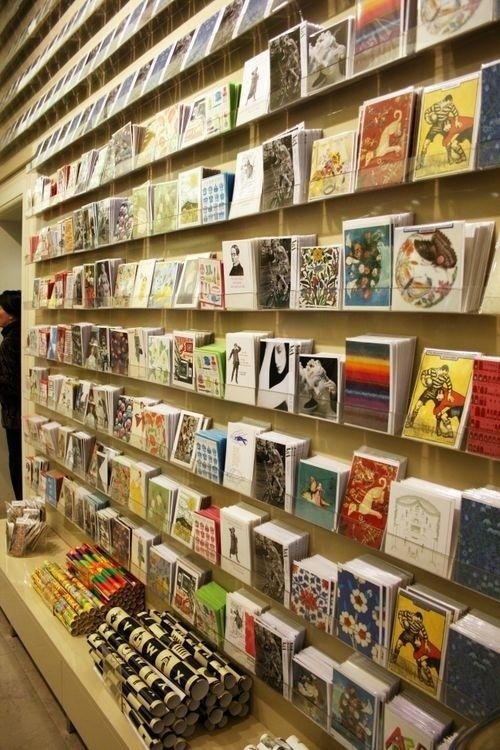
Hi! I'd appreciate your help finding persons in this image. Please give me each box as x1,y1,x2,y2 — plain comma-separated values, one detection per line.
0,289,23,501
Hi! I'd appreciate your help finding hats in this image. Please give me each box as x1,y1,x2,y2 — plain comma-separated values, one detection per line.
0,290,21,318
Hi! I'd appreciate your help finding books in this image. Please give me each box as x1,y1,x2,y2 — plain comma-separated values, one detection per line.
23,0,499,314
22,321,500,750
